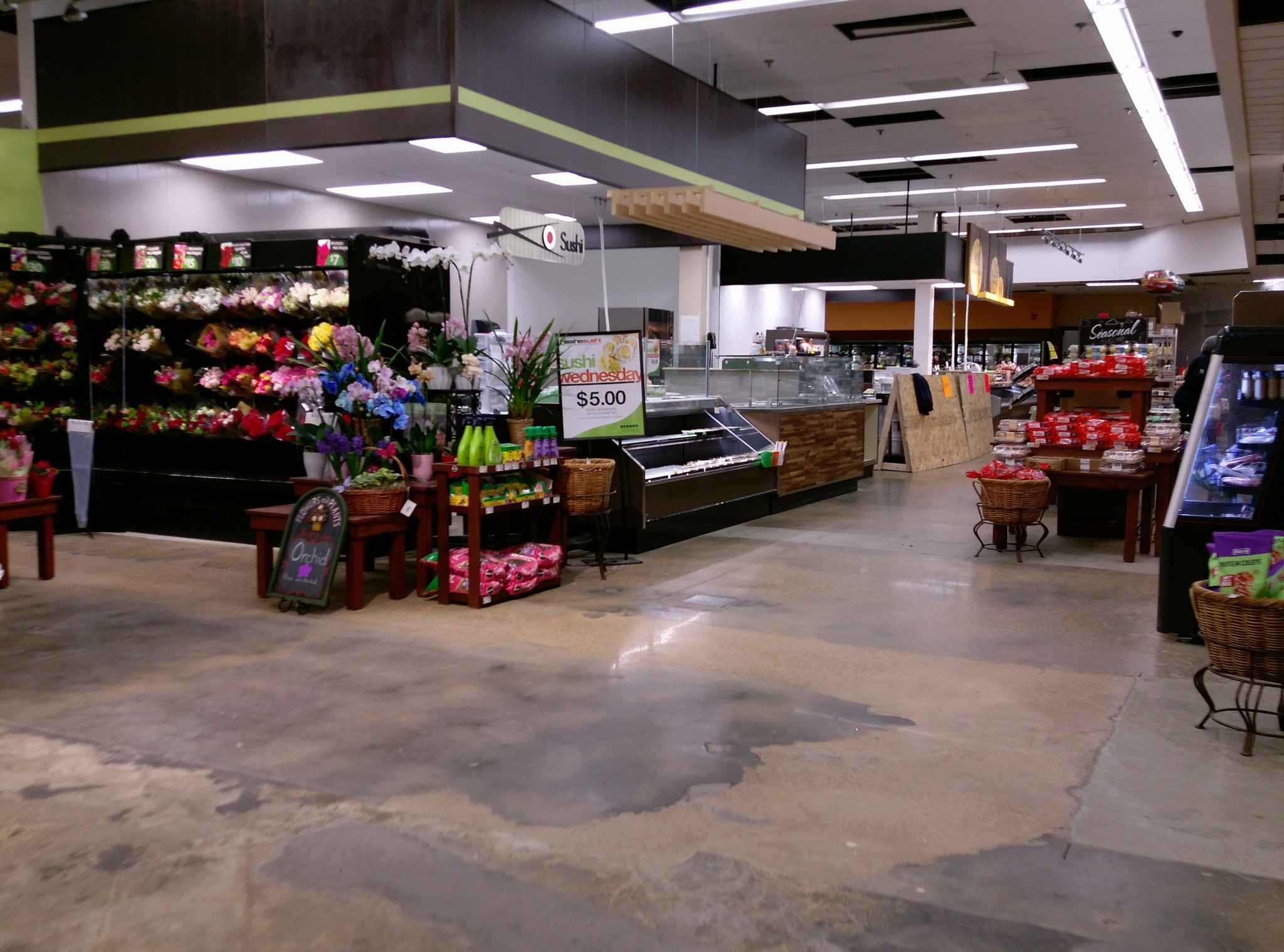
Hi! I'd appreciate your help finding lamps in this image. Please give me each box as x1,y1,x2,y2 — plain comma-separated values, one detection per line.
1040,213,1085,263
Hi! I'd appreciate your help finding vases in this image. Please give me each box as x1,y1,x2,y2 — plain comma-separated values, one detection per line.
33,476,55,497
452,389,482,414
507,419,534,444
0,475,29,501
426,367,452,390
454,374,472,389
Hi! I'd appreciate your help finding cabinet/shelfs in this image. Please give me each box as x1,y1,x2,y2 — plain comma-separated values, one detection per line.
992,324,1183,563
432,446,576,608
0,226,450,546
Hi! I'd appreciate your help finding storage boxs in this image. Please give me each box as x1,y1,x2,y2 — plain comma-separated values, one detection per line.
1068,456,1104,471
1026,456,1066,471
1156,324,1175,334
1158,302,1185,325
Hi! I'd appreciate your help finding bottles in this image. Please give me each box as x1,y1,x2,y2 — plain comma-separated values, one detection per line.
1241,370,1284,400
457,425,502,468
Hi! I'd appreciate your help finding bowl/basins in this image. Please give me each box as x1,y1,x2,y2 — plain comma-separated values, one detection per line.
647,384,670,399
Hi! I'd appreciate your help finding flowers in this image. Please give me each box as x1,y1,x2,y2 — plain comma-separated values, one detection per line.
0,241,574,494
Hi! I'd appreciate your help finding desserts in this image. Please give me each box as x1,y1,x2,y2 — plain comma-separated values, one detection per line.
603,333,639,362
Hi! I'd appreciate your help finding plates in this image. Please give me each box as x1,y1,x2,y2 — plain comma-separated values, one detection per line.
682,426,741,436
1220,477,1262,486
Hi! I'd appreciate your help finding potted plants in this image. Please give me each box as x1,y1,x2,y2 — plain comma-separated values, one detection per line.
286,417,336,479
406,422,439,480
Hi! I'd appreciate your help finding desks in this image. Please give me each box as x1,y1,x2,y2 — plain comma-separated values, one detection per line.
0,495,63,589
246,504,407,611
290,477,436,597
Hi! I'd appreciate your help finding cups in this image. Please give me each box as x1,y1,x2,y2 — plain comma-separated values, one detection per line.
761,451,773,468
772,452,779,466
777,452,785,466
